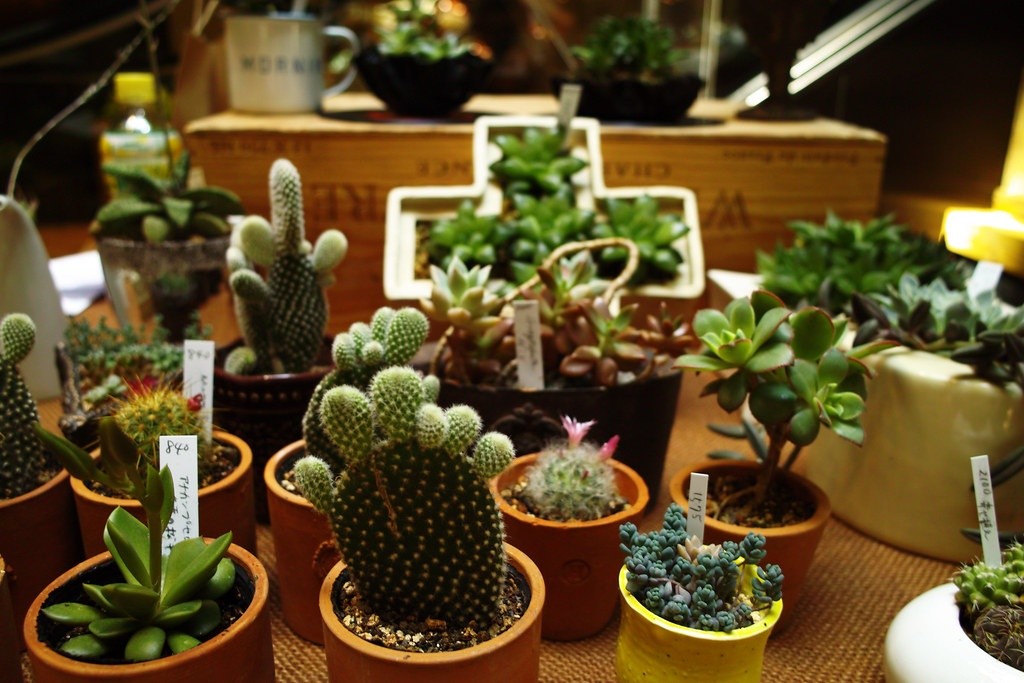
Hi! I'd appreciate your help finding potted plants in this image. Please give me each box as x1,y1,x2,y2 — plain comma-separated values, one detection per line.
0,0,1024,683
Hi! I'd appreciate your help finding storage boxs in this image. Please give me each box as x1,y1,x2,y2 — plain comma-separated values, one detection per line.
183,91,891,342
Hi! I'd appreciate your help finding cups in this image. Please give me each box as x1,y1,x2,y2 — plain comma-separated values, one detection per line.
225,15,358,114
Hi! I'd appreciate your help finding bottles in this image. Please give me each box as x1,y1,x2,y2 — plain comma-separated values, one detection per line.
99,72,187,201
